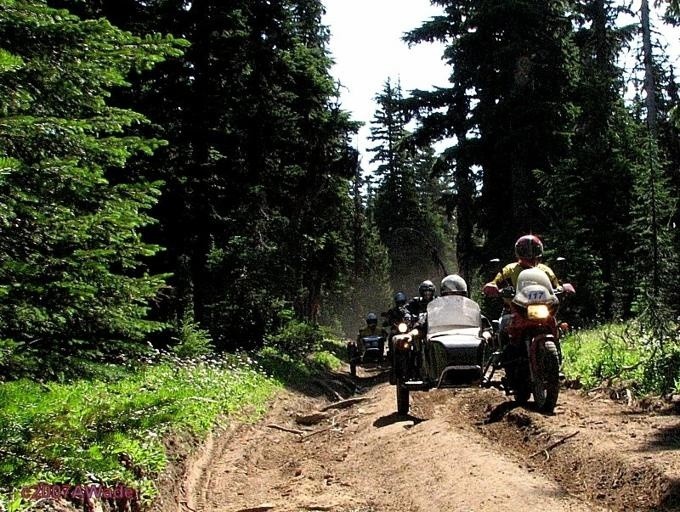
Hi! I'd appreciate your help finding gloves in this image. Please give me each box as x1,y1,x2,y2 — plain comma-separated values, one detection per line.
562,284,576,297
484,284,499,296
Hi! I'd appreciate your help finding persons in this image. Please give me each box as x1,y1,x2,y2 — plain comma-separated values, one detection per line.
387,274,483,348
358,313,388,341
483,235,568,348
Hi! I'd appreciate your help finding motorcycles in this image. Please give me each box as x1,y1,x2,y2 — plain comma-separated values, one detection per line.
347,276,497,413
487,256,579,413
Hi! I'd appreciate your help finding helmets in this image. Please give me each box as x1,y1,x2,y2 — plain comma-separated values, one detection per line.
395,292,407,303
514,234,544,268
419,280,435,296
440,274,468,296
367,313,378,324
